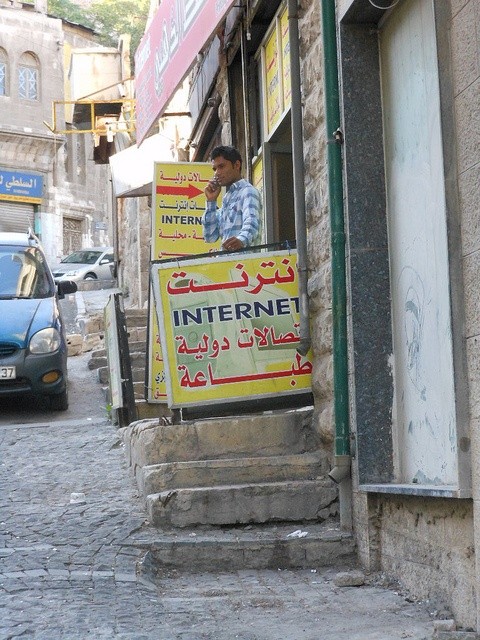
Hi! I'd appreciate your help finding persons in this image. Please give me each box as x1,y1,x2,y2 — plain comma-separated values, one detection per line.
201,146,262,253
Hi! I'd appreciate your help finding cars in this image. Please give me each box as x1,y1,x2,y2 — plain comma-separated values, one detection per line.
52,247,114,290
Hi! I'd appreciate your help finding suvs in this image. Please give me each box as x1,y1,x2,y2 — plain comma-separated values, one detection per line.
0,227,77,410
